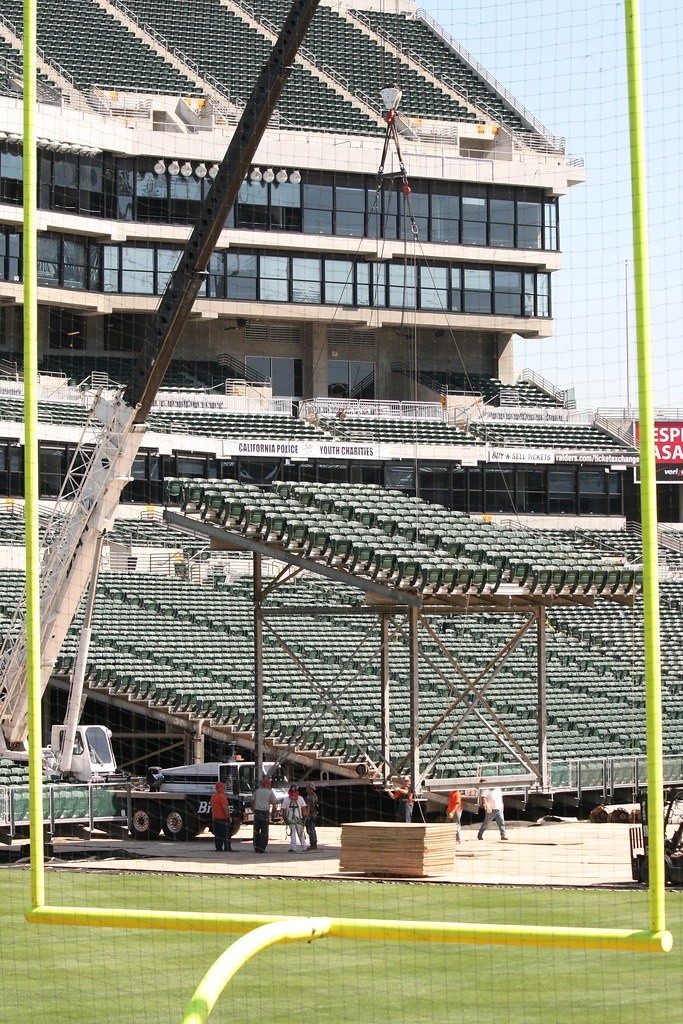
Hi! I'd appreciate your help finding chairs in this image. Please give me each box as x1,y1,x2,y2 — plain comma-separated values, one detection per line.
0,350,683,823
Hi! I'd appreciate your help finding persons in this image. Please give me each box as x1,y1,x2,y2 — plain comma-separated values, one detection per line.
305,782,319,850
386,776,414,823
281,784,310,852
447,791,463,843
252,779,276,853
478,787,508,840
210,782,232,852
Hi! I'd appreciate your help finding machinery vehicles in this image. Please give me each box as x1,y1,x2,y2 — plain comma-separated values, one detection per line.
0,0,419,785
115,761,290,842
629,791,683,887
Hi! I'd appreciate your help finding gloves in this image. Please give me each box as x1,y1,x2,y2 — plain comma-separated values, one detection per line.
449,812,454,819
483,803,491,813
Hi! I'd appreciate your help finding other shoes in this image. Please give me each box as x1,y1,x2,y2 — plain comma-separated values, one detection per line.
501,837,508,840
307,846,317,850
477,834,483,840
288,849,295,852
303,849,309,852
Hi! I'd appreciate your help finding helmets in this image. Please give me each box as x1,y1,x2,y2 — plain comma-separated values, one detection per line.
307,782,316,788
290,784,298,792
263,779,270,787
216,783,224,791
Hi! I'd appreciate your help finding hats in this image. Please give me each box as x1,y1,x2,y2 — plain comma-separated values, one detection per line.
403,776,410,783
